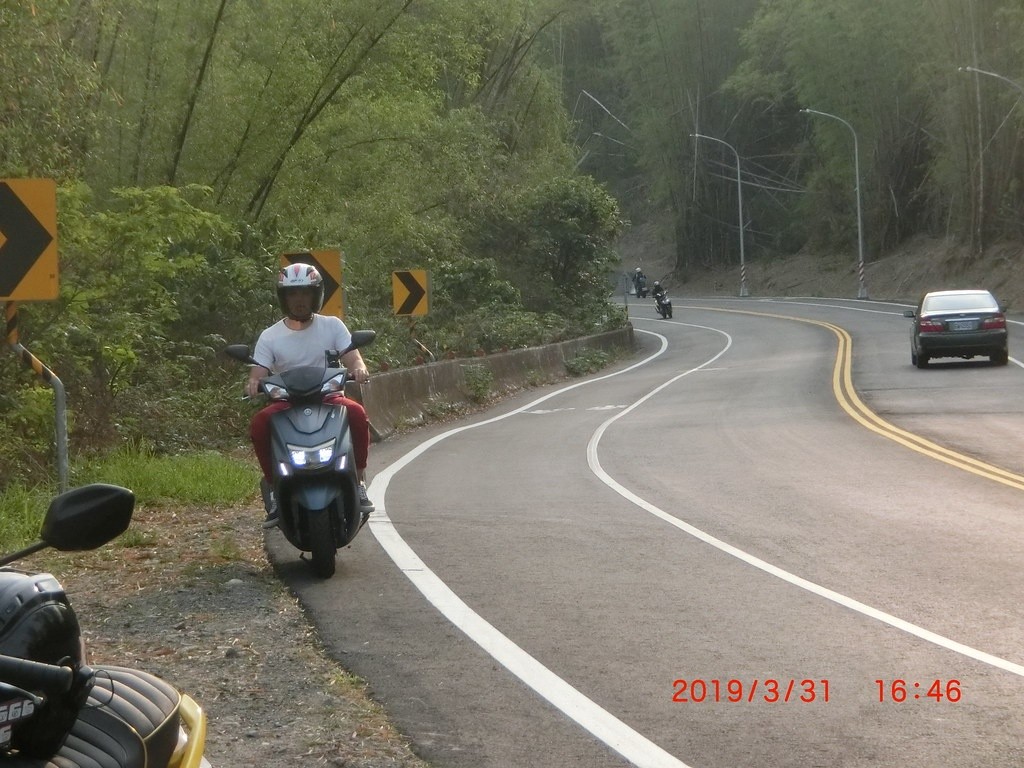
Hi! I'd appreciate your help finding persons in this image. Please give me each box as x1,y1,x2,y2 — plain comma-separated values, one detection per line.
652,280,667,297
631,267,647,281
244,262,376,529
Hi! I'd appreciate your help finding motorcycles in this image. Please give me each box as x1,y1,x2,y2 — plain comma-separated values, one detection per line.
224,329,377,578
653,289,672,319
0,483,213,768
632,277,649,298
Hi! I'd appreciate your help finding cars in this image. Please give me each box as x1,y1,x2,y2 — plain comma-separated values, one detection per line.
903,289,1008,368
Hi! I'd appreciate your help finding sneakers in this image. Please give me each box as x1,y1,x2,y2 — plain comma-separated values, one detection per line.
262,490,279,529
358,481,375,512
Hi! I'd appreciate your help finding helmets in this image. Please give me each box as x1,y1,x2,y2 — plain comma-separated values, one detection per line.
635,267,642,272
654,281,660,287
1,564,99,768
278,262,324,291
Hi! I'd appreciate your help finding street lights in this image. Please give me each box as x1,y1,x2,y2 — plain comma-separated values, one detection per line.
799,108,868,300
689,133,750,297
